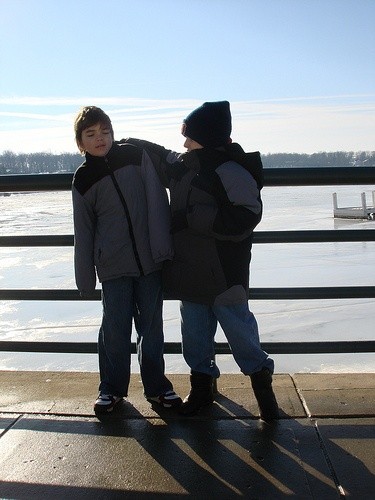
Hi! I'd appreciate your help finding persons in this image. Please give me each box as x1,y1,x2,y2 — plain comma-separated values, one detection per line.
71,105,184,412
114,100,279,433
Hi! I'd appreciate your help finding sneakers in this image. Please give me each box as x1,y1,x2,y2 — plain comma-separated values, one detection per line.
144,390,183,408
94,392,123,412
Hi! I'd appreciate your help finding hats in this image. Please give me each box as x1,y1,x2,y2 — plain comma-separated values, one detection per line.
183,101,232,147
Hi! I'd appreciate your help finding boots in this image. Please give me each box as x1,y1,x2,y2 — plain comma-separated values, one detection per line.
250,371,278,423
183,374,214,409
